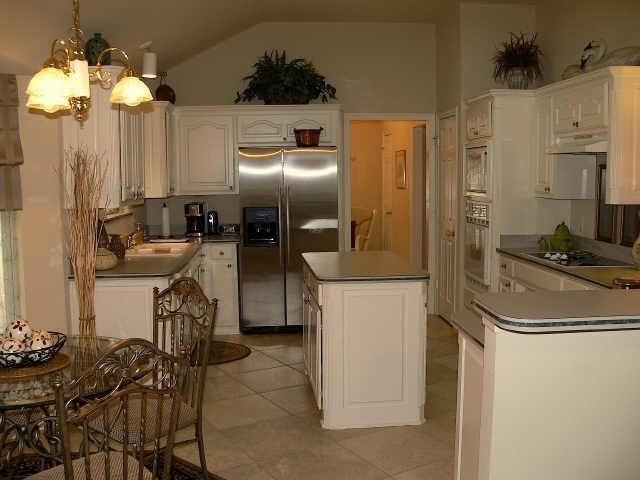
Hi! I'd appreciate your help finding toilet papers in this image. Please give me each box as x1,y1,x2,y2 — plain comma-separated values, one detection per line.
161,207,171,237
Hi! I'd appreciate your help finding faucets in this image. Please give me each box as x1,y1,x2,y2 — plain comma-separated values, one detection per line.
127,233,133,247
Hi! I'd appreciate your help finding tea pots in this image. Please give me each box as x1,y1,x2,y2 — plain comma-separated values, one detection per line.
551,221,573,252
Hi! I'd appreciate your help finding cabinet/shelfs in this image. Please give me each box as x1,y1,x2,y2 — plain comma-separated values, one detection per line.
68,242,240,396
61,66,145,211
303,260,428,431
238,105,340,149
544,70,608,153
498,257,610,294
533,65,596,199
146,100,238,198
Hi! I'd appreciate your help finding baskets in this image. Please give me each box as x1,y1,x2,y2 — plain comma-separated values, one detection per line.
0,331,67,368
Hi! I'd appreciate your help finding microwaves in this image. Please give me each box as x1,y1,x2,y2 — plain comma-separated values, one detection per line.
464,148,488,194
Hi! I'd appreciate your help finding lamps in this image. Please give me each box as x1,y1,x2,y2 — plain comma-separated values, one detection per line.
141,51,158,80
24,0,155,121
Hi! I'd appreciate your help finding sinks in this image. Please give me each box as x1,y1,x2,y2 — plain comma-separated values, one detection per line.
134,243,195,248
124,249,186,256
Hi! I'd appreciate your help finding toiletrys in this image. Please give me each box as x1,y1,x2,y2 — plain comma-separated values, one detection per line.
134,222,143,244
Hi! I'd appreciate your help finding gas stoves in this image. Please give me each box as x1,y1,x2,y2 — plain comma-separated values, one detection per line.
524,250,638,270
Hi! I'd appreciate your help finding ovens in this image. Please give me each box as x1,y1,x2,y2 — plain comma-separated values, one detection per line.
465,200,489,289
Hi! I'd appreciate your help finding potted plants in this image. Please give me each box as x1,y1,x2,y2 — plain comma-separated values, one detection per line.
236,46,339,105
489,30,545,89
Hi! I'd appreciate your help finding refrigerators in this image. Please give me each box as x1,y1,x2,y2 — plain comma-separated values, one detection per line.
237,145,338,335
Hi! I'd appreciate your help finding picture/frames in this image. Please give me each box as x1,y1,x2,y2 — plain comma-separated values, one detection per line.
395,150,406,189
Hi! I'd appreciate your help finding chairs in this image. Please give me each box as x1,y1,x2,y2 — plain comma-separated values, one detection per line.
24,276,218,479
355,209,377,250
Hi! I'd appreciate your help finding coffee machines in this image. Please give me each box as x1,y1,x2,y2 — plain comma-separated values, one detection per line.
184,202,205,237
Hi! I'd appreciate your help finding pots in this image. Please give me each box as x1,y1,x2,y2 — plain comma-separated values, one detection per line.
294,128,321,147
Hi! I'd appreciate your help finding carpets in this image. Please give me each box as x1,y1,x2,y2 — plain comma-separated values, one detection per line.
189,341,251,366
